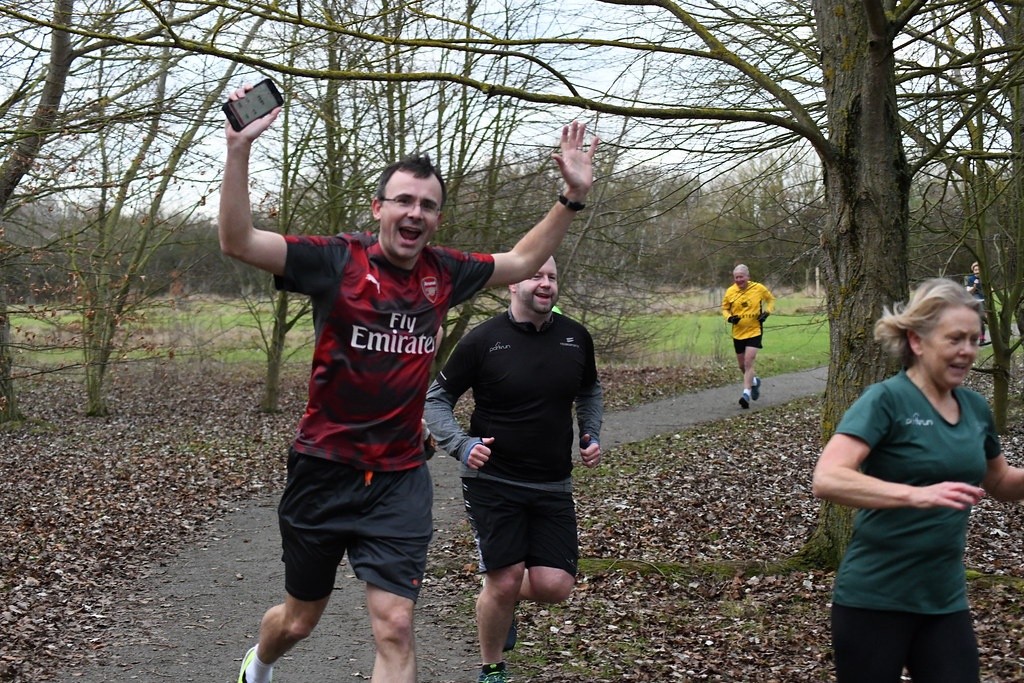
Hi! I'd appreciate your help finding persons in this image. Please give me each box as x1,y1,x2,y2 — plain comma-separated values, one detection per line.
965,261,992,347
721,264,776,409
217,87,602,683
422,253,606,683
810,276,1023,683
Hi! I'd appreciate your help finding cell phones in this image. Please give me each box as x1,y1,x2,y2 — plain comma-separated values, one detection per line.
223,78,283,131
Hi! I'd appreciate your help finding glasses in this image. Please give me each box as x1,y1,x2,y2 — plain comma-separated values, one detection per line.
378,195,442,219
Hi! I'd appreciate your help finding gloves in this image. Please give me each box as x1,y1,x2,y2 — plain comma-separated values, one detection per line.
757,311,769,323
727,315,741,325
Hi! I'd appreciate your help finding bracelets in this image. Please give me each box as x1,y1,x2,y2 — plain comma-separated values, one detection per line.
559,193,586,213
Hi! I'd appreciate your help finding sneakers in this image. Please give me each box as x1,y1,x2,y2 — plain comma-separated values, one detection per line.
501,613,520,652
752,377,760,400
235,643,257,682
740,394,748,410
475,666,507,683
